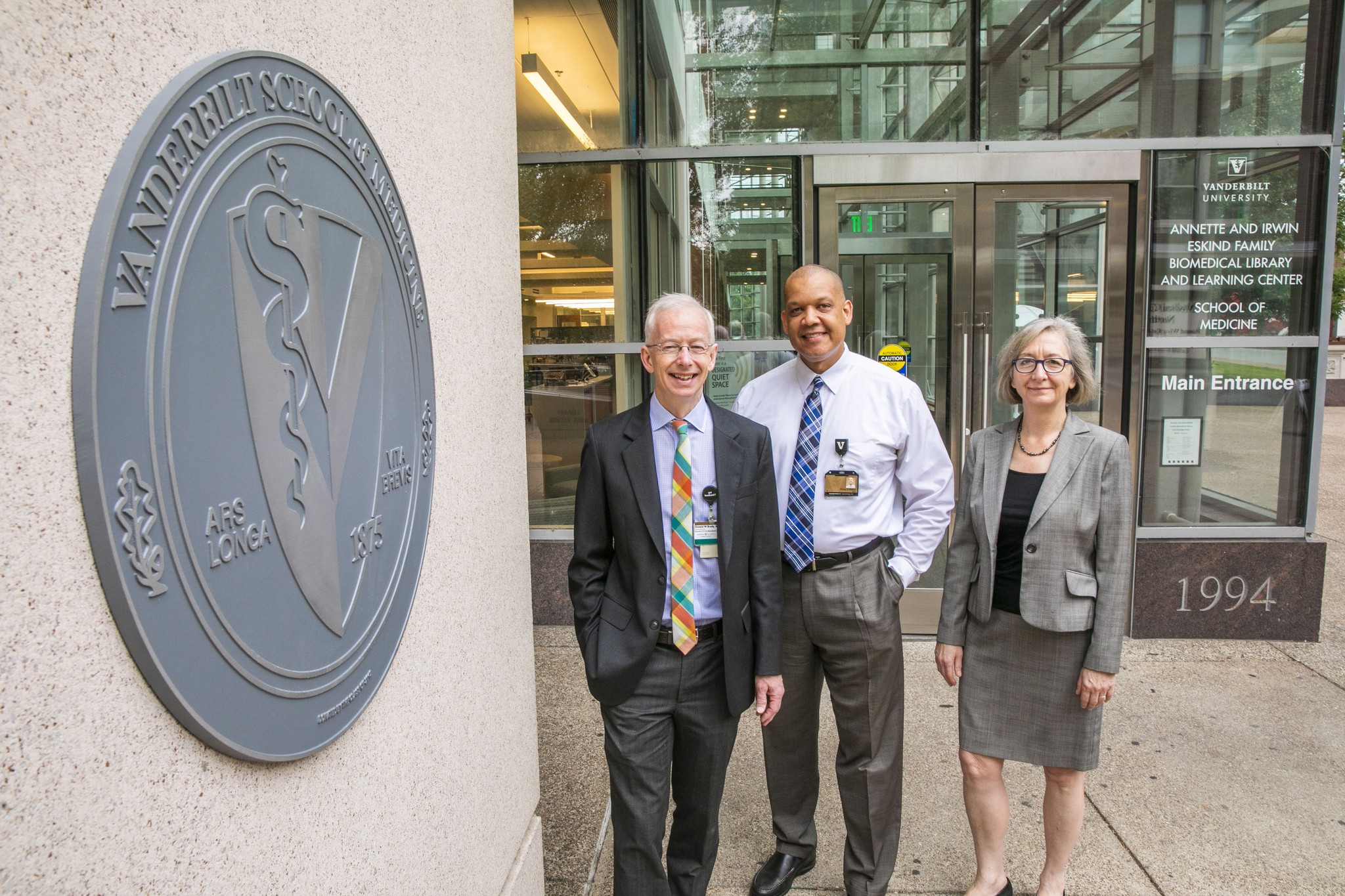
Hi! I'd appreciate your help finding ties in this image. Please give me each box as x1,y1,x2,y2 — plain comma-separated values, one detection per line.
784,375,825,573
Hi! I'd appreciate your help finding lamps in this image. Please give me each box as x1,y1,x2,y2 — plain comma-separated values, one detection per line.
522,17,599,150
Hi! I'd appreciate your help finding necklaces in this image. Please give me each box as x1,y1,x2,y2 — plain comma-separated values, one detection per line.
1017,421,1061,456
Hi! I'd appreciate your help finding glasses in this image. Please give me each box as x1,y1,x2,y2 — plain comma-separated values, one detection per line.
647,340,713,354
1011,358,1072,374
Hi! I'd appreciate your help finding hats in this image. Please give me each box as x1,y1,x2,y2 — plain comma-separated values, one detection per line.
668,419,696,656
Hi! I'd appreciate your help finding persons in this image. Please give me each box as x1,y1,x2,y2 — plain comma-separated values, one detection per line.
726,264,955,896
567,291,784,896
934,314,1135,896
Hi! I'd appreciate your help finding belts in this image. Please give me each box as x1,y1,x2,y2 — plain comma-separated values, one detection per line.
657,619,723,646
784,534,886,572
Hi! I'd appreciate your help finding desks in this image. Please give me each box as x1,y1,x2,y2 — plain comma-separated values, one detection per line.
525,372,612,497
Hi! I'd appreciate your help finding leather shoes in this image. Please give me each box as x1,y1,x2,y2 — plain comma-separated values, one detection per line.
749,851,809,896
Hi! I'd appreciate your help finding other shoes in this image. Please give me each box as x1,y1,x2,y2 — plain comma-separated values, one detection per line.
995,877,1013,896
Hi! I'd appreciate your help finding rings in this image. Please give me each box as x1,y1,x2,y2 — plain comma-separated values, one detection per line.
1099,695,1106,698
